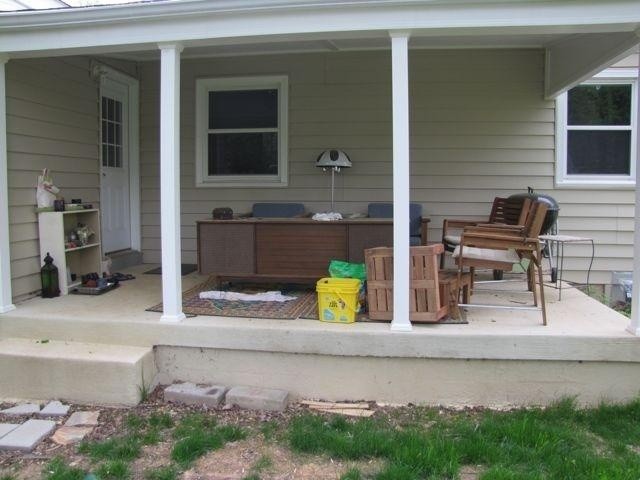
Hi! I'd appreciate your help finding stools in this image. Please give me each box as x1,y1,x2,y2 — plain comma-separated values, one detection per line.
438,267,472,319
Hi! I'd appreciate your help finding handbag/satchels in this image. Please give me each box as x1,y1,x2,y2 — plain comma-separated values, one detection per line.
36,166,57,208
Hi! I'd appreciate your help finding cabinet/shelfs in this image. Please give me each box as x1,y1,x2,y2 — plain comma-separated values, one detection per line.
36,206,104,295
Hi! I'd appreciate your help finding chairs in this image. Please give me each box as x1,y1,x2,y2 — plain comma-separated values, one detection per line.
254,202,305,217
435,196,595,325
364,243,450,324
370,201,424,247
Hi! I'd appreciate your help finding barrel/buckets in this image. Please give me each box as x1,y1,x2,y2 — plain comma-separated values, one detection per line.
316,278,361,323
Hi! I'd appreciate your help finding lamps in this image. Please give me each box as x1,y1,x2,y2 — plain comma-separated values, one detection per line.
39,252,62,298
315,146,352,218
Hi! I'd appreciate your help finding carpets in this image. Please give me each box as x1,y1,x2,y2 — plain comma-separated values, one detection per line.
144,283,321,320
141,263,197,276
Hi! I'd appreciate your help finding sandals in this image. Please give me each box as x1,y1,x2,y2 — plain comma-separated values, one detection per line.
112,271,136,281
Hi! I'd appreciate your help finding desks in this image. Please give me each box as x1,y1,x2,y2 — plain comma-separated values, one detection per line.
194,211,430,292
